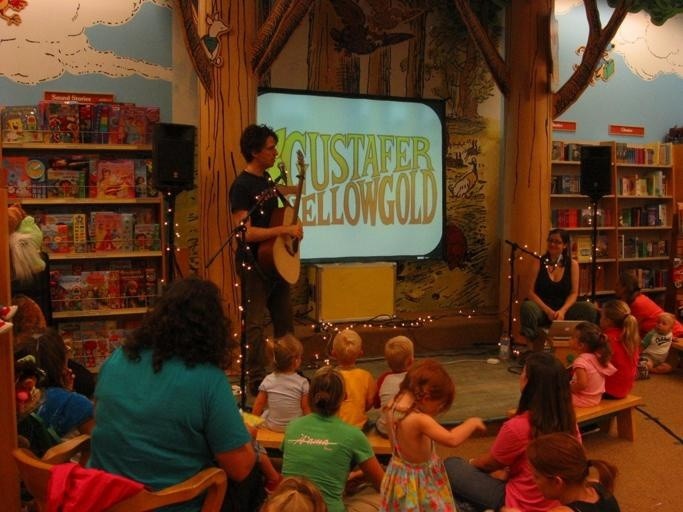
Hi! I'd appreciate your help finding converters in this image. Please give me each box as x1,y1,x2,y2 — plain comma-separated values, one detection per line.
487,358,498,364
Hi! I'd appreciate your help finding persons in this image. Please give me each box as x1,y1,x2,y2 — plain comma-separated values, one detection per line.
440,350,587,511
225,121,313,399
87,278,266,510
0,250,99,503
557,320,617,408
577,299,642,437
639,311,680,381
514,227,601,367
523,433,621,511
252,329,490,512
613,269,683,342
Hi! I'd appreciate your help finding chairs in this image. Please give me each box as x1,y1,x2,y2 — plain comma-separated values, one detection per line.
11,431,229,511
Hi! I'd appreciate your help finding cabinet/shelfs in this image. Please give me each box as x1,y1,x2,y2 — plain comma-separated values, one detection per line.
549,137,676,297
0,143,167,320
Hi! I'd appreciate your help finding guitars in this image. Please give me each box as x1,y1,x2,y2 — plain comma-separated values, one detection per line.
256,149,305,284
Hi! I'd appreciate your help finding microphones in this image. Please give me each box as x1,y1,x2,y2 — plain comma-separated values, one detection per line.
277,162,288,184
554,254,563,268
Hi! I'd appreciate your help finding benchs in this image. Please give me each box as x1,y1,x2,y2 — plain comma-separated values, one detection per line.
254,426,392,459
503,394,649,444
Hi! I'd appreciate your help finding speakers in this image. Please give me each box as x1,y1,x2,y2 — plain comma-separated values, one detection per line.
580,146,612,195
151,123,194,191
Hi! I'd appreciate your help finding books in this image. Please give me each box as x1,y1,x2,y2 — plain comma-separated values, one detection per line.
60,320,151,375
2,152,160,199
44,269,163,313
30,213,163,254
1,99,160,148
551,138,673,294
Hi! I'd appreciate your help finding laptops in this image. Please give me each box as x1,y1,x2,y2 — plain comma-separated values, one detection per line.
541,320,580,341
